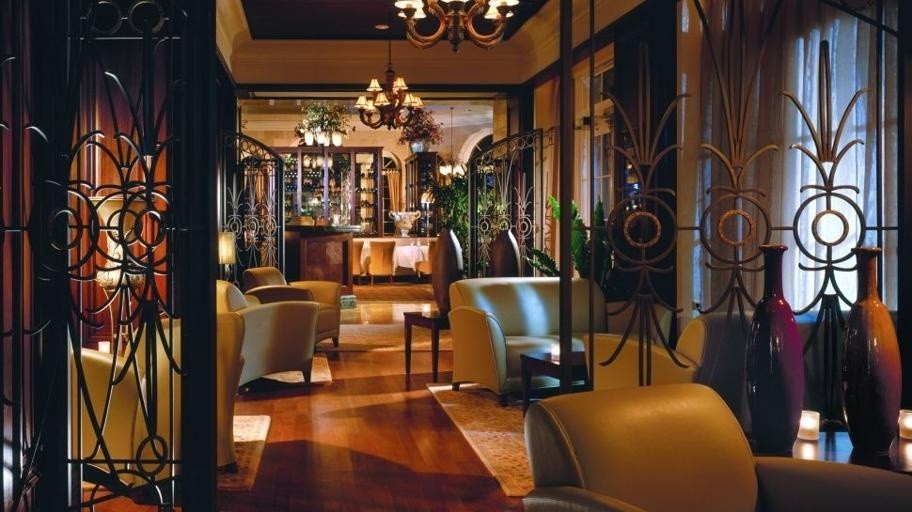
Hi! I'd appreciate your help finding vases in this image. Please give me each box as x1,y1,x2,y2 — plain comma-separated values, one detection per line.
431,227,466,312
743,244,802,446
491,229,522,279
842,246,903,454
324,127,335,147
410,139,426,153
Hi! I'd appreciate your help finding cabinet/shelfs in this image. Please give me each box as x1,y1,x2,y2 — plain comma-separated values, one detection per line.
267,145,449,307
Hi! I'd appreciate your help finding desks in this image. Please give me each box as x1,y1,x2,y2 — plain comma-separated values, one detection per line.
400,310,451,379
520,344,589,414
747,431,912,476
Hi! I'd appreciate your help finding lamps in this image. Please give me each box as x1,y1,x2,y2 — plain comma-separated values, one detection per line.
440,105,469,179
392,0,521,56
76,190,157,358
354,1,425,130
218,231,237,264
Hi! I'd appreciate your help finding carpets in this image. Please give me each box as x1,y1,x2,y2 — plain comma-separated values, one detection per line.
259,350,333,387
314,320,456,354
79,413,273,493
424,379,536,497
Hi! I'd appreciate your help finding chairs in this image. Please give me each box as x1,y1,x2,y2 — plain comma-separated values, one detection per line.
216,281,319,394
69,309,245,493
520,381,912,512
243,267,343,353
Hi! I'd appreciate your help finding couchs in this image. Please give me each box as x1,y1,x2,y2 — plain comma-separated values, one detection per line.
445,276,670,407
581,305,900,455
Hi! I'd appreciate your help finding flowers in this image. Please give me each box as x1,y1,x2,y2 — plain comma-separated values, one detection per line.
302,100,354,147
397,108,444,145
